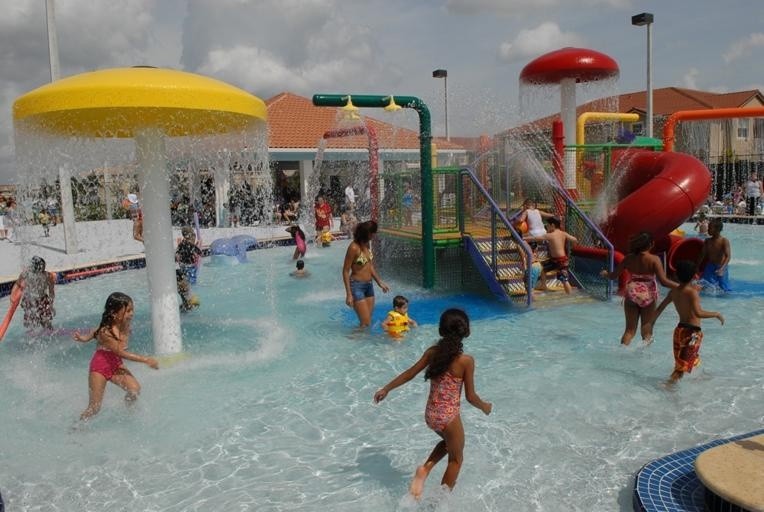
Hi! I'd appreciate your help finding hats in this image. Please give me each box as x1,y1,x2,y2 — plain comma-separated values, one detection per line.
127,194,138,204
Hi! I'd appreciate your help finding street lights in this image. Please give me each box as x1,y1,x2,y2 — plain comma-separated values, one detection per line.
433,69,450,141
631,12,654,138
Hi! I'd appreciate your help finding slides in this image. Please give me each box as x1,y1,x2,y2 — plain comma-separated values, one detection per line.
591,148,711,292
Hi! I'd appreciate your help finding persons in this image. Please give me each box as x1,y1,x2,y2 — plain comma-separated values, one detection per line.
601,231,700,344
641,260,723,385
521,217,577,293
342,220,389,340
223,180,416,279
695,213,709,234
726,173,762,217
699,220,730,292
73,292,158,419
0,185,216,331
374,308,492,502
517,198,554,266
382,296,418,339
